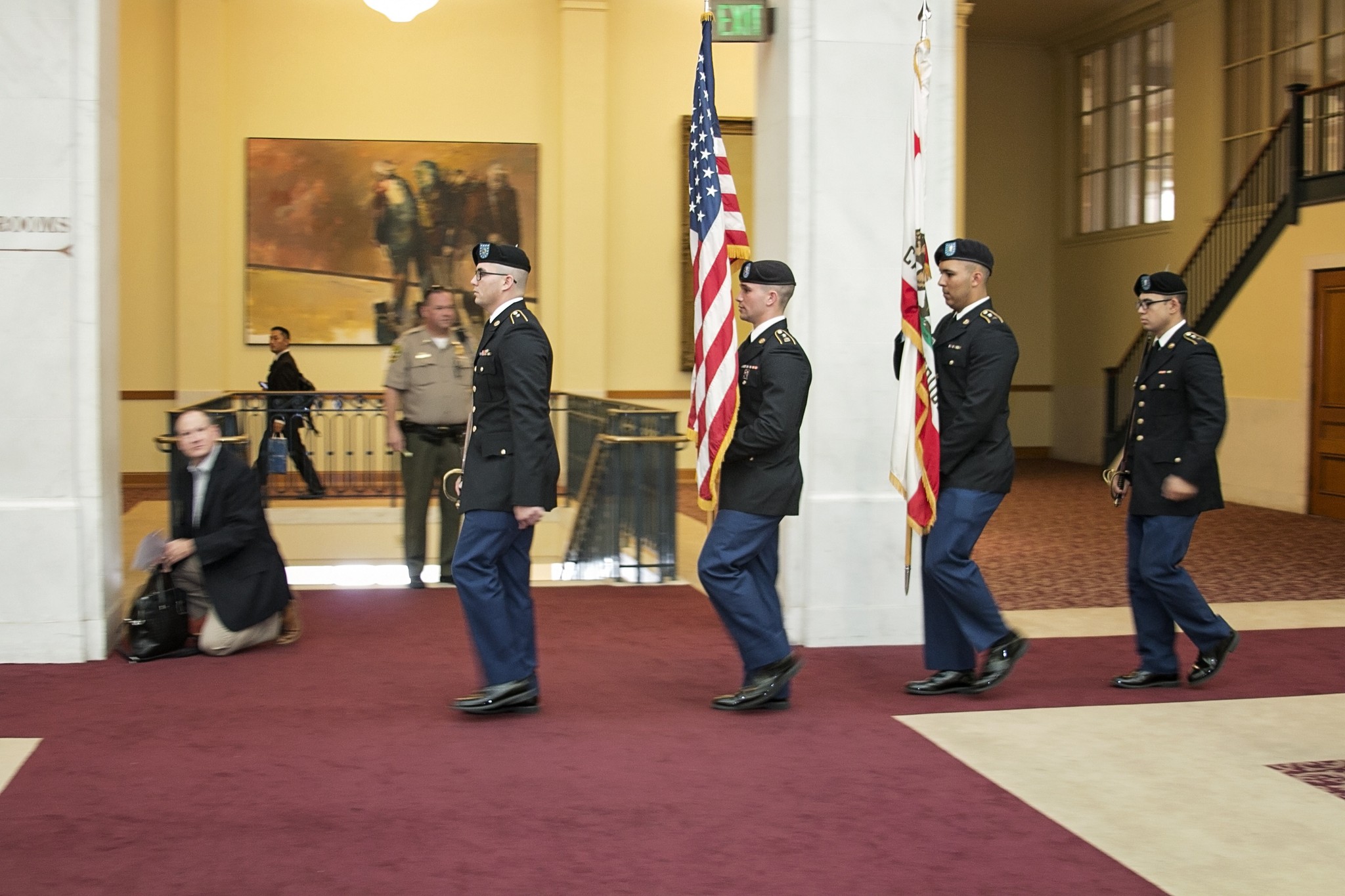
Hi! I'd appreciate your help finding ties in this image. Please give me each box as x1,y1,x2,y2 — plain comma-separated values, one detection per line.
1144,340,1160,376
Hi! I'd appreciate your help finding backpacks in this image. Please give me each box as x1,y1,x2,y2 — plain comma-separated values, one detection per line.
281,359,320,435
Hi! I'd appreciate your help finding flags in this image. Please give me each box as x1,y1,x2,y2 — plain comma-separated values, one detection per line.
684,13,753,515
889,36,941,533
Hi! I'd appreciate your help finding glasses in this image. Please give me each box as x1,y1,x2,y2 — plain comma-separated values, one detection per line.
425,284,454,291
1135,299,1173,310
474,270,517,284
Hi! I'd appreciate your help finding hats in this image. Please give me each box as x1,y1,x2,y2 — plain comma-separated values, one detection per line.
472,242,531,273
739,260,796,286
1133,271,1188,297
934,238,993,277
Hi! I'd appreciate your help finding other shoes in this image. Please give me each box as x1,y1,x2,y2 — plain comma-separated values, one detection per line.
298,488,323,499
274,599,303,645
440,574,456,583
411,575,425,589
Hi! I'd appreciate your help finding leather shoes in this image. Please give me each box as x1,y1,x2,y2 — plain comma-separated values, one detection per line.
465,696,537,713
710,694,789,710
451,673,539,711
974,632,1024,692
905,669,975,695
1188,629,1238,685
1110,668,1181,687
733,651,799,708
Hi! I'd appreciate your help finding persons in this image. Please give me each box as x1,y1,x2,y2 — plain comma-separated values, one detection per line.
696,259,813,711
893,238,1029,695
382,286,475,590
448,241,560,715
148,409,302,656
1107,271,1242,688
362,156,520,338
249,326,326,500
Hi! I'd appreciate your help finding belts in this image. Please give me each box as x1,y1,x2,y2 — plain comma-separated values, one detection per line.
400,419,468,438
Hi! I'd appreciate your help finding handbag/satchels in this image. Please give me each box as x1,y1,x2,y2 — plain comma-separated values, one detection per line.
268,431,288,475
118,566,204,663
